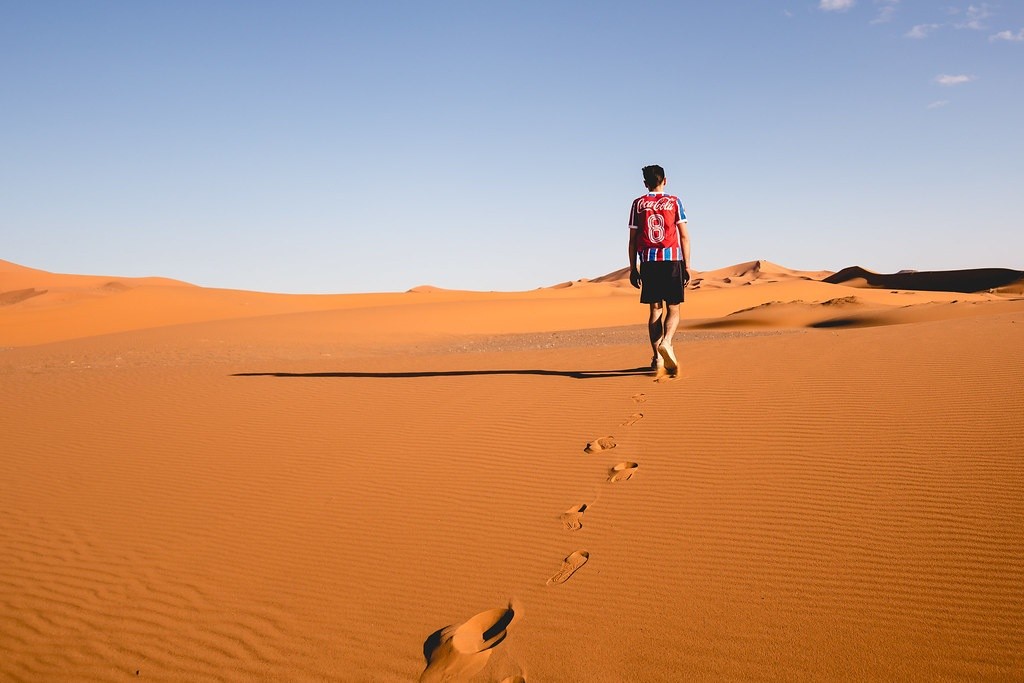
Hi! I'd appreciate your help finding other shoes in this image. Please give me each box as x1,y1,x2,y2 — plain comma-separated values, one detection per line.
658,342,678,374
651,358,663,369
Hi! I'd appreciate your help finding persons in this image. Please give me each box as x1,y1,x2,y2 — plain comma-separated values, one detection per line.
628,165,690,374
